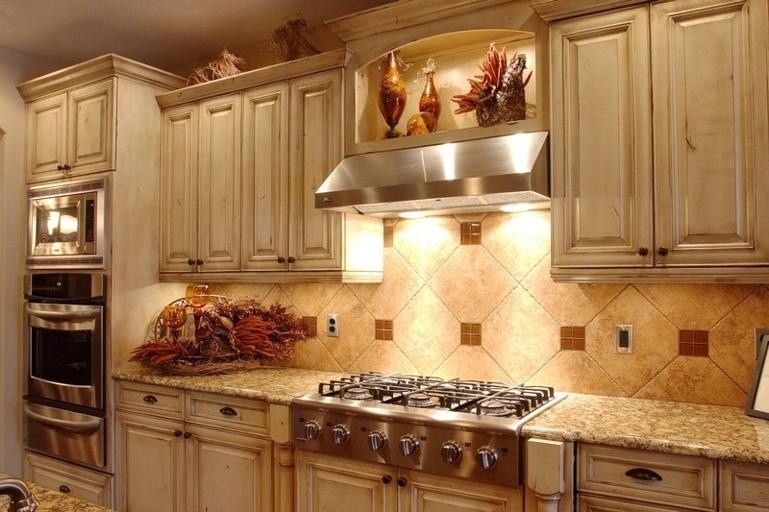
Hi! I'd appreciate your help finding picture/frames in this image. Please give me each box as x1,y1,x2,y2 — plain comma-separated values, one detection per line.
744,334,769,422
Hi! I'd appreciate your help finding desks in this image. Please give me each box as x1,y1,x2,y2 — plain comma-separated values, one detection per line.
0,468,113,510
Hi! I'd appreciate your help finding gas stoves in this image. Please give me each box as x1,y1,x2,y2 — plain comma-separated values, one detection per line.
290,368,569,490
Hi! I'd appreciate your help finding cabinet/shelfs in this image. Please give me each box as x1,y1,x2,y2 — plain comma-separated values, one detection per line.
536,1,769,289
574,439,769,511
241,49,383,283
20,448,113,507
153,70,244,285
294,446,523,511
18,54,120,181
117,377,272,512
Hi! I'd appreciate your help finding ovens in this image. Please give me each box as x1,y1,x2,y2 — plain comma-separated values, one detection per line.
17,269,107,473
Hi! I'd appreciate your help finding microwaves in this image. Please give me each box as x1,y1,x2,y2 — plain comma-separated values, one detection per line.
23,191,101,260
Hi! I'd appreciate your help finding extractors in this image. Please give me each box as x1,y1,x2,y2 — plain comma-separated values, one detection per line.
315,128,551,219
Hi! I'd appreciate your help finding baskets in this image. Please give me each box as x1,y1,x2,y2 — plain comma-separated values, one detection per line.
154,294,261,377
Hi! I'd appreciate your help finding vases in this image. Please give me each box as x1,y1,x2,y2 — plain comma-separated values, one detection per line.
377,51,408,128
419,70,443,135
